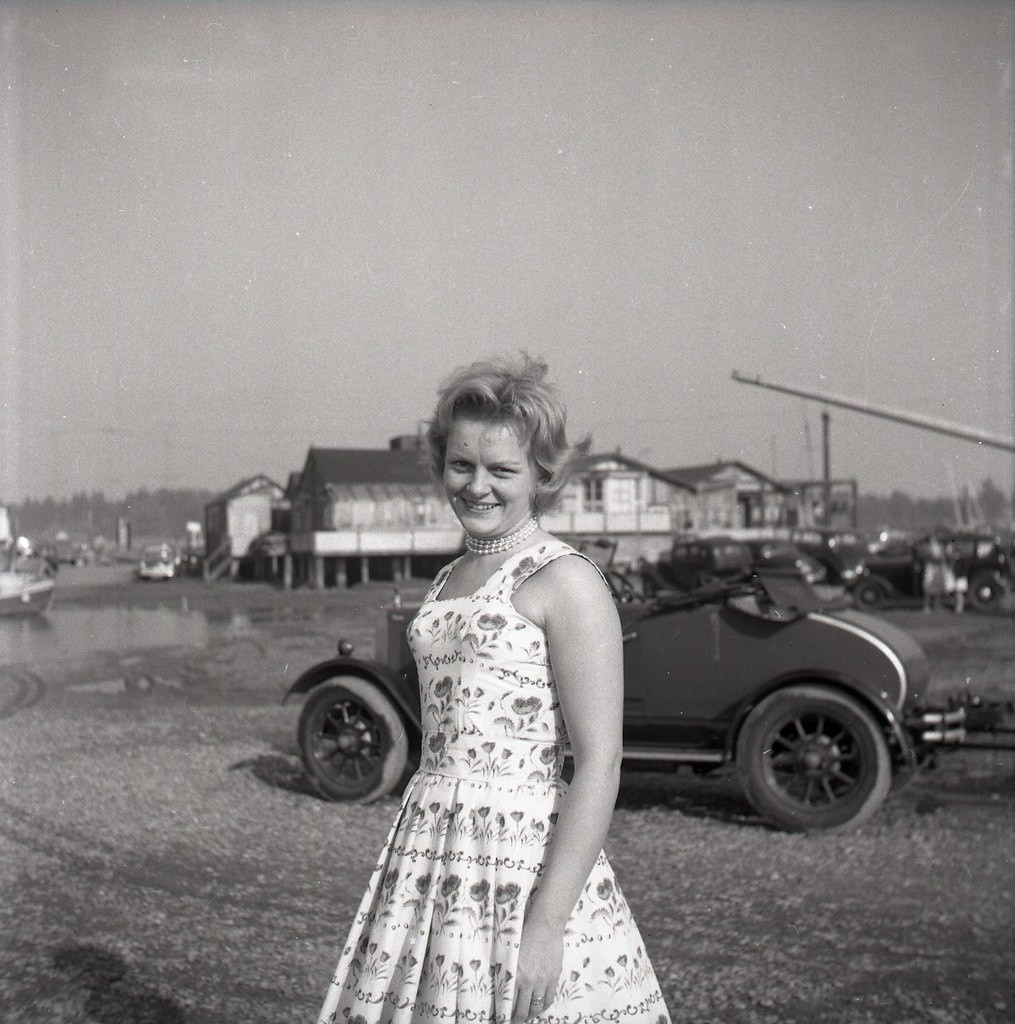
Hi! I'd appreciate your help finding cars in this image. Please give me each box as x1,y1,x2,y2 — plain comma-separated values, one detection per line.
279,536,934,837
851,531,1008,611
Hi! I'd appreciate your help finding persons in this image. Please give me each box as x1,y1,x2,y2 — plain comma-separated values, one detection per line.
313,355,672,1024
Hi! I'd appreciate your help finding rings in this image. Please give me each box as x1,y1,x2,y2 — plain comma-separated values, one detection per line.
531,997,544,1004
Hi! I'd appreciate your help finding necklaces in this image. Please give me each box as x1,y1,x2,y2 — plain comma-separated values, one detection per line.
465,514,538,555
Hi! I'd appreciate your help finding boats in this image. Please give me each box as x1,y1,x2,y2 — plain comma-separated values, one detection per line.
0,570,58,619
